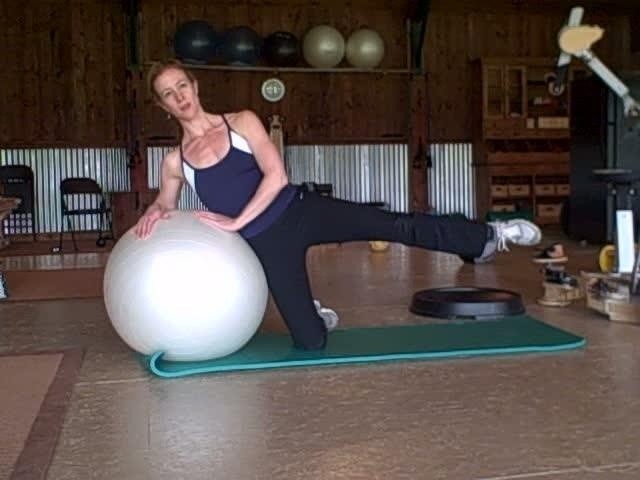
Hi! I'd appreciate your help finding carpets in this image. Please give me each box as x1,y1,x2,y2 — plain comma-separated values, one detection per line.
0,342,88,479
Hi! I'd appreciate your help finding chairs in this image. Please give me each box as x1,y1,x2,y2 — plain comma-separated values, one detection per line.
1,164,37,243
54,176,112,252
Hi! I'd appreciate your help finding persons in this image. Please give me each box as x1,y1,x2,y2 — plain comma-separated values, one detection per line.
133,58,544,351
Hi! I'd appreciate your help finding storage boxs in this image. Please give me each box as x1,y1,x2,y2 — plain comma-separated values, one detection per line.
491,183,570,218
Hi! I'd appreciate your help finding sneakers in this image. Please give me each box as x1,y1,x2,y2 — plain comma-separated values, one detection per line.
542,266,577,289
532,242,568,265
487,219,543,247
312,298,340,331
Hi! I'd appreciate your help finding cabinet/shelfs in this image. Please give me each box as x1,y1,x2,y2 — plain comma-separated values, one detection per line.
475,51,575,232
